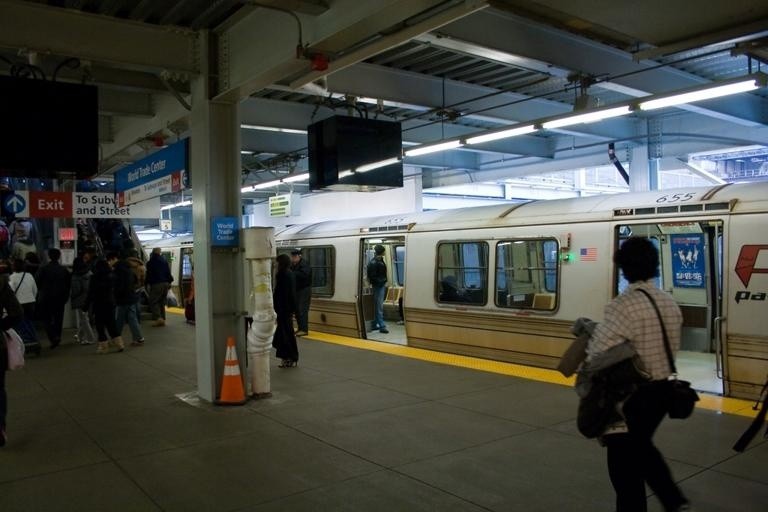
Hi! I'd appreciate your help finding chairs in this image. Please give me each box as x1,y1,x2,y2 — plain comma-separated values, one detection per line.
534,294,555,310
384,287,403,320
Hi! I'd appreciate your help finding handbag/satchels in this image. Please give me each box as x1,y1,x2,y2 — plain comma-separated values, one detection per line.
275,330,299,360
1,325,26,372
662,375,699,418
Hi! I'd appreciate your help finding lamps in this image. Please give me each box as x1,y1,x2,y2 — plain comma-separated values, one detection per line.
160,199,192,211
239,172,309,195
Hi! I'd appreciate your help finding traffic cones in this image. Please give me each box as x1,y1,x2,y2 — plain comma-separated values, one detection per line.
211,334,251,407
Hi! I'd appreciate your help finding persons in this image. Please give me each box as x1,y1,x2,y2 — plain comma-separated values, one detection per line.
438,275,473,302
0,273,25,446
272,252,300,369
365,244,390,333
289,250,313,336
584,234,690,511
0,217,176,352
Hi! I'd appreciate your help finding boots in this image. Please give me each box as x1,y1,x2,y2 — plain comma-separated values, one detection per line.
96,341,110,354
112,335,125,350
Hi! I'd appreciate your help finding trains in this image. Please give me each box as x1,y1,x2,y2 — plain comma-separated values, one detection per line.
138,173,768,406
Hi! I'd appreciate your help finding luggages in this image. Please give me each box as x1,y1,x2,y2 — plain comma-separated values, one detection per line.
184,298,194,320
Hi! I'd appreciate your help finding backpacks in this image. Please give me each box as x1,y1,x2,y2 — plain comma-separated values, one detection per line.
368,258,386,287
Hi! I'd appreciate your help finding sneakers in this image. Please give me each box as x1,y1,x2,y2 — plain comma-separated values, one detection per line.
379,326,389,334
129,337,144,345
294,329,308,336
151,318,166,327
48,335,62,347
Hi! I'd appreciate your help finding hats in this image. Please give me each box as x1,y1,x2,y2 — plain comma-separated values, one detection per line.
290,250,304,255
128,248,138,257
47,248,60,261
374,245,385,255
277,254,291,266
152,247,161,255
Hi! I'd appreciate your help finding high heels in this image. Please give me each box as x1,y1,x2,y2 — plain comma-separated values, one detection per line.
277,358,298,368
